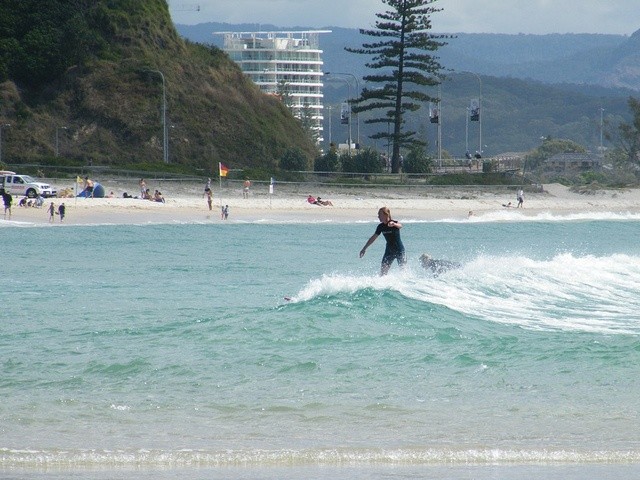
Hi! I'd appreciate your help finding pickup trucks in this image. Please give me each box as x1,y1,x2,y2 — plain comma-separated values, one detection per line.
0,175,52,198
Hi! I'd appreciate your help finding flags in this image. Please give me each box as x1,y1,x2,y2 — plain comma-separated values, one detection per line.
219,162,229,176
77,176,84,183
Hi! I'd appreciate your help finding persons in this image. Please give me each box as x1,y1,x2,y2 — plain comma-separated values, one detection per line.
242,176,250,200
139,179,146,199
110,191,133,198
516,186,524,209
418,253,464,280
359,206,406,279
19,195,37,208
47,202,57,222
203,178,211,199
207,188,213,210
58,203,65,224
221,204,229,221
3,191,12,215
308,195,334,206
144,189,165,203
83,177,93,199
